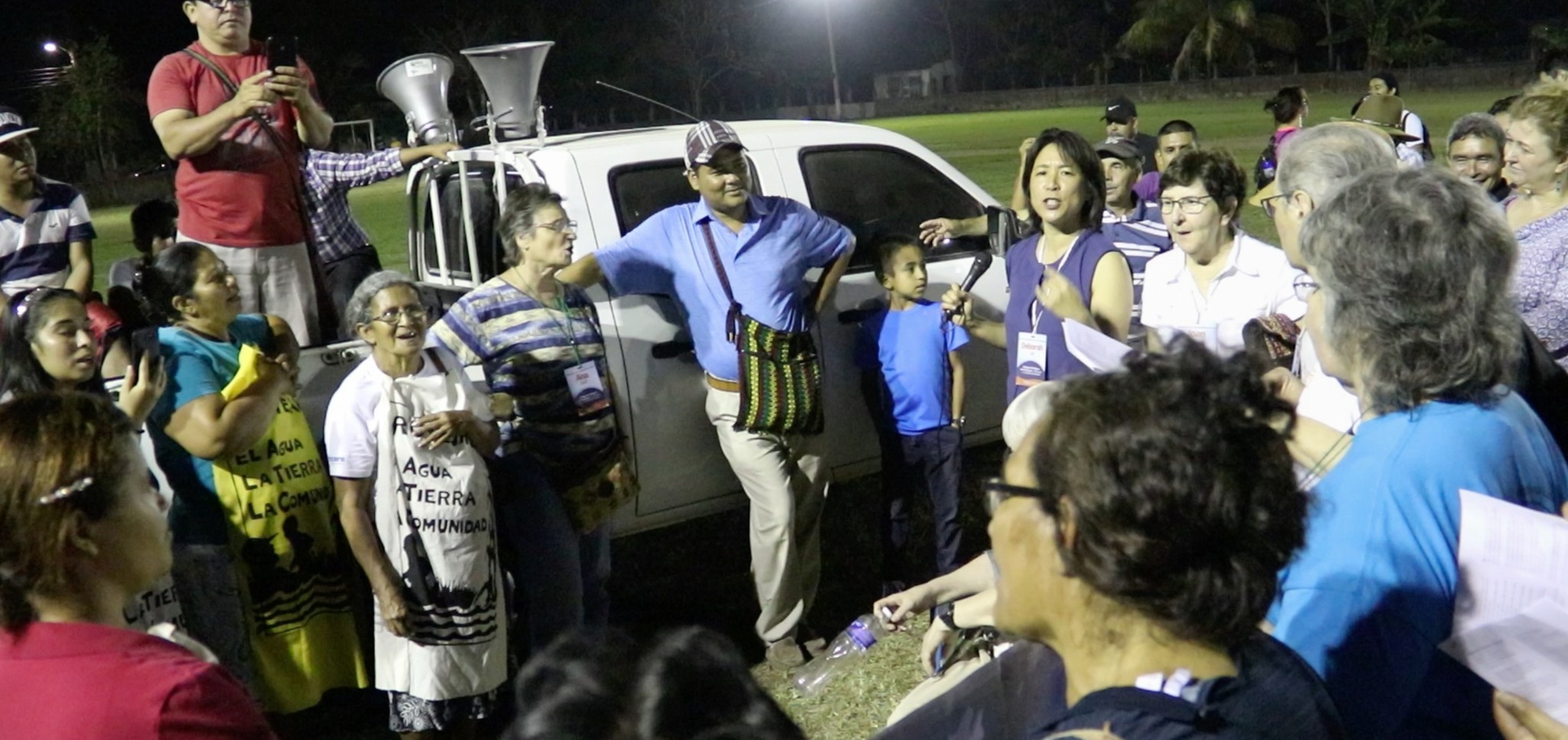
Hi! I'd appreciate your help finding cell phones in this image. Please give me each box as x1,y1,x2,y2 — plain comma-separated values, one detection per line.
265,33,296,93
131,324,159,387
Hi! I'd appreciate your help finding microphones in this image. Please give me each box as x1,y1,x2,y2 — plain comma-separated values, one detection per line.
942,249,993,323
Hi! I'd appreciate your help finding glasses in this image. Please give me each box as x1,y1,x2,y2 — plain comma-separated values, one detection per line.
1293,274,1320,302
200,0,251,8
1261,194,1289,218
369,304,430,322
1156,194,1220,214
979,476,1047,512
533,218,579,234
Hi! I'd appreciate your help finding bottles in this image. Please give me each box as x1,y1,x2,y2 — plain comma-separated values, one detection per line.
792,605,894,698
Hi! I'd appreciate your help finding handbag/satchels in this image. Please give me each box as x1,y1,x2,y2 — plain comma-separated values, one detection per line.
552,427,641,539
727,314,825,433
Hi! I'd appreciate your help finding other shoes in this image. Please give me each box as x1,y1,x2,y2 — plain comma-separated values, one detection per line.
796,620,828,655
762,632,808,673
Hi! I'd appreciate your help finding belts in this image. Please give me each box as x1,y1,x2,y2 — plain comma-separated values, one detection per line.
706,373,740,392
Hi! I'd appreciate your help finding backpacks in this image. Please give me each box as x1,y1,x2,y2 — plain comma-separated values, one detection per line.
1254,129,1294,194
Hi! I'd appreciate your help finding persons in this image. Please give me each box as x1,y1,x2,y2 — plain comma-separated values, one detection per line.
1,1,1568,740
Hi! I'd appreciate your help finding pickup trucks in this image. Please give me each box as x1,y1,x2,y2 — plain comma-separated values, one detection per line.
110,115,1035,546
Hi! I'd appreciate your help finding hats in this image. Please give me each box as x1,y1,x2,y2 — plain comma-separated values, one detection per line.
0,105,40,144
1093,136,1142,160
1330,95,1421,141
1099,95,1138,120
682,120,748,176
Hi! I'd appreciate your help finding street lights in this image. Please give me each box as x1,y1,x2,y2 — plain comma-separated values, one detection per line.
45,42,79,70
